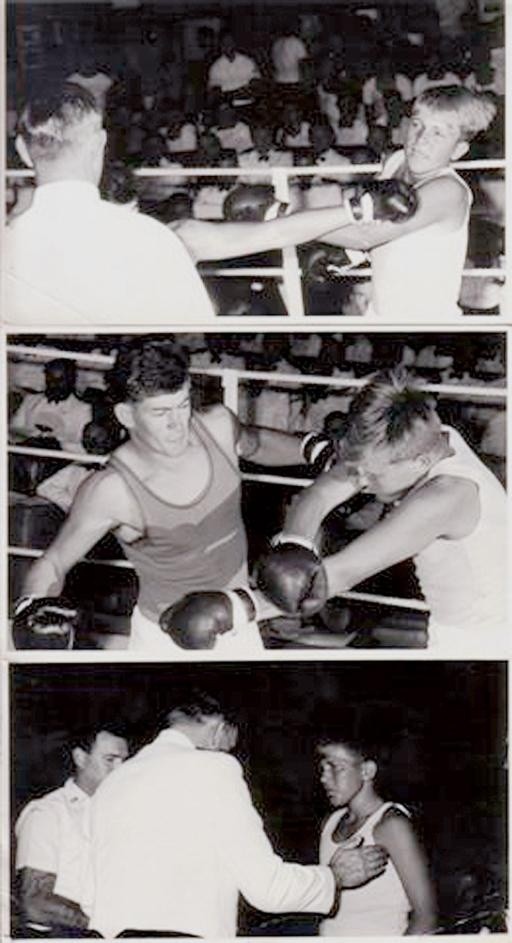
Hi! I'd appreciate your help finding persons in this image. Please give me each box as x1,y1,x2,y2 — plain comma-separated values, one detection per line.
1,0,505,325
13,719,130,937
54,685,391,936
7,329,507,655
314,733,438,935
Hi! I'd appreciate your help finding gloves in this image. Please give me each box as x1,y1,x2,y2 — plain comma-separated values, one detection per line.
303,241,367,281
345,179,417,225
302,429,366,517
257,536,330,618
160,589,257,649
11,595,77,650
223,187,290,222
